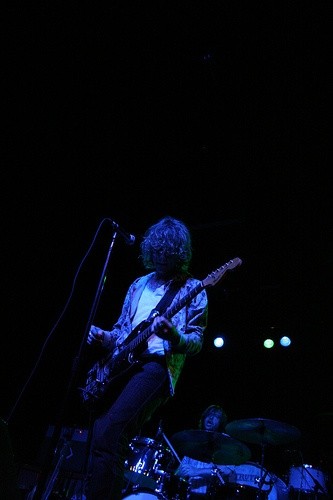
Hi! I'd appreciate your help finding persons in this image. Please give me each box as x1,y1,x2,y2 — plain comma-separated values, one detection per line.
87,217,207,500
167,405,235,477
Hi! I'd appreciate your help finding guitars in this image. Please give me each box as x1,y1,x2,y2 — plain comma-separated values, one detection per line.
78,257,245,411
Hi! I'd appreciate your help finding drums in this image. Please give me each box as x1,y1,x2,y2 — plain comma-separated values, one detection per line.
120,488,170,500
125,436,173,489
215,462,290,500
287,464,328,493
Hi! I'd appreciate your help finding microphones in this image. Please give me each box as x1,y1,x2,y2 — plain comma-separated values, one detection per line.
112,221,135,245
154,425,162,440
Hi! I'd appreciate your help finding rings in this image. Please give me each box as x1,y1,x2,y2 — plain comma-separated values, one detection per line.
89,333,92,336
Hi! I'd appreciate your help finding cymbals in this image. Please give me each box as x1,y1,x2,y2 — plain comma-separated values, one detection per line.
224,417,301,447
170,428,251,466
